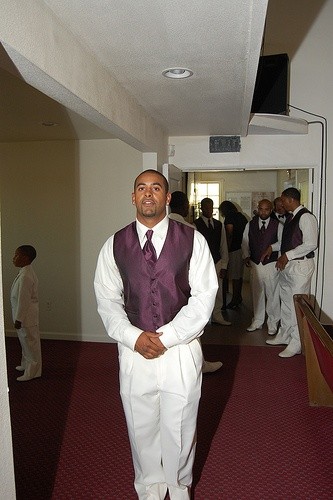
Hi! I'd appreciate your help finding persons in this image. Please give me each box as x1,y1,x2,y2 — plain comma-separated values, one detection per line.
193,198,231,325
260,188,318,357
271,197,290,223
218,201,248,309
11,245,42,381
93,170,219,500
169,191,223,373
241,199,284,335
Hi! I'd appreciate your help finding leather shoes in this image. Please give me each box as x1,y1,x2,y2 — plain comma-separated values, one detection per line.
212,317,232,326
16,375,34,380
267,329,277,335
202,361,223,374
279,348,296,358
245,326,262,331
16,366,25,371
265,337,289,345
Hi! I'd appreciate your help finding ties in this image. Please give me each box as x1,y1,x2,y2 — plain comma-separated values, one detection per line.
142,231,157,264
208,219,213,229
287,213,293,222
261,221,265,231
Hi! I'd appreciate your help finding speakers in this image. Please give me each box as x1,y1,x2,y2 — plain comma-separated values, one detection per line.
250,52,290,116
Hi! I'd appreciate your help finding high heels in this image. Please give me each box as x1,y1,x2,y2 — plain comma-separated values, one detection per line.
228,297,242,308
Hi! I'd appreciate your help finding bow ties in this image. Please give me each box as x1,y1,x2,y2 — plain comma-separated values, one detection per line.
278,215,284,218
